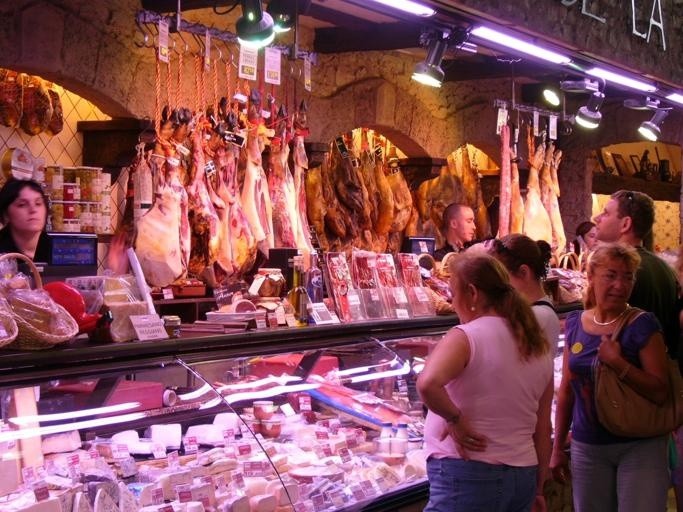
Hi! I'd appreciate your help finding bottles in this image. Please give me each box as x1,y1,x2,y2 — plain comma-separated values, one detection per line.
287,250,322,326
381,423,409,461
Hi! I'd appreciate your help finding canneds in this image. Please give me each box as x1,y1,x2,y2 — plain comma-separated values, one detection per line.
46,165,112,234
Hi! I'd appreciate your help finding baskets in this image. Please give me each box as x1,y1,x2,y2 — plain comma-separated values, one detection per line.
0,253,79,352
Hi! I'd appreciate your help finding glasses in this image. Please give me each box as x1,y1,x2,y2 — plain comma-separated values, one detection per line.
627,191,641,207
491,235,529,261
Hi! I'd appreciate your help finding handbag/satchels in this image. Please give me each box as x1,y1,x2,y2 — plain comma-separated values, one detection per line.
597,356,682,438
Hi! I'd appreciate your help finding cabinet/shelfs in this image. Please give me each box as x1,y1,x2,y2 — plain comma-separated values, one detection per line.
7,299,585,507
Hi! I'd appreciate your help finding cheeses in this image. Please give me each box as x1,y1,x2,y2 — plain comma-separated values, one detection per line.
86,277,149,343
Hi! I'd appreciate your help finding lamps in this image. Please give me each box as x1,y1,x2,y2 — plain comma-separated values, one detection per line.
542,69,673,142
412,27,451,90
236,1,275,49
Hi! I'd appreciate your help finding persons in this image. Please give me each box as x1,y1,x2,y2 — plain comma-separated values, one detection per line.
572,221,598,257
415,253,554,511
548,241,672,512
433,203,499,260
487,234,561,352
0,180,52,267
594,190,682,364
